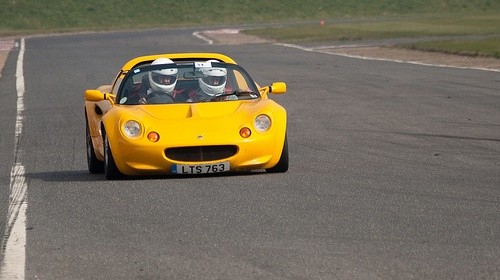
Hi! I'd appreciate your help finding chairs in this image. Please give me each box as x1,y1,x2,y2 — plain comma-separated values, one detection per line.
131,74,152,104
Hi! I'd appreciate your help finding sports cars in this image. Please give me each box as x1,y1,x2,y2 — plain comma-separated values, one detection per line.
84,52,290,180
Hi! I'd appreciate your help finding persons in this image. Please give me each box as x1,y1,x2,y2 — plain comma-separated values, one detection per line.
183,59,238,104
128,58,186,106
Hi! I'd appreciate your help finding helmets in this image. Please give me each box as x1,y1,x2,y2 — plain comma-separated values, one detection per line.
198,60,227,96
149,57,179,92
